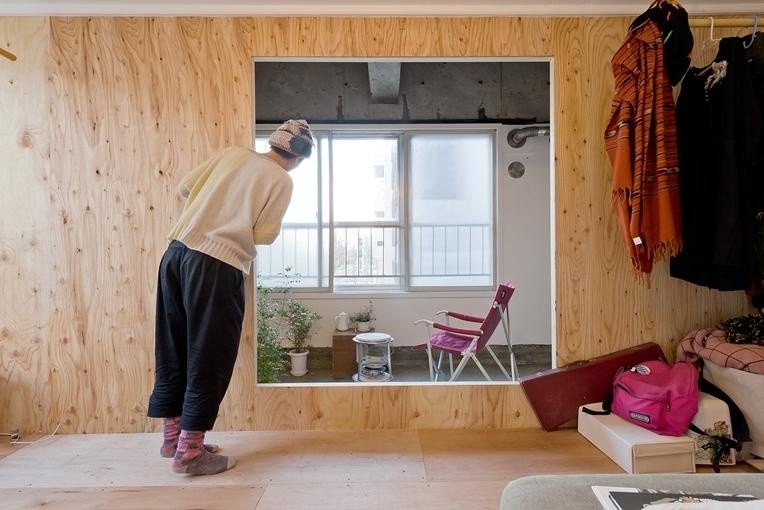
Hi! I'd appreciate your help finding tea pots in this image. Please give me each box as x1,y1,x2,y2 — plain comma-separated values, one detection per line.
334,311,351,331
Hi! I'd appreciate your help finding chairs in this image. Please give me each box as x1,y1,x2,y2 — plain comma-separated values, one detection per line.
411,282,520,382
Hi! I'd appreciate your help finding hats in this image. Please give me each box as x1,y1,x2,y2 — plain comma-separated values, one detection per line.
268,119,314,158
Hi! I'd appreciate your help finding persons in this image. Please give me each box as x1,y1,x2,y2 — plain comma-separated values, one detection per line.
147,118,314,479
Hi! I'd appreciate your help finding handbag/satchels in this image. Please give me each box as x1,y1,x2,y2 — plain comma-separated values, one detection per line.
602,357,699,437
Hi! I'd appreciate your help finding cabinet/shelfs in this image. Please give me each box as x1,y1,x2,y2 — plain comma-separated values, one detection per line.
331,325,375,381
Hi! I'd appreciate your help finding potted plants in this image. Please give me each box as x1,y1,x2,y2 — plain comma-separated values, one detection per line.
276,296,323,377
349,300,376,332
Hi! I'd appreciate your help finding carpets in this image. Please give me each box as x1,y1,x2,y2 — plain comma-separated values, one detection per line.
499,467,763,510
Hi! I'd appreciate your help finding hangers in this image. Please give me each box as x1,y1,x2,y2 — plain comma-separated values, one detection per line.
692,14,722,78
739,15,757,49
